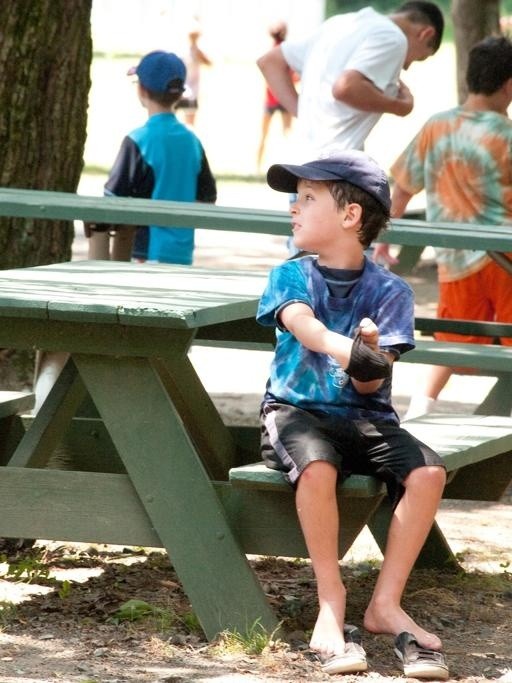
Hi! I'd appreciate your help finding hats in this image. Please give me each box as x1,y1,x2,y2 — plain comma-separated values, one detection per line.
265,148,393,218
125,48,187,96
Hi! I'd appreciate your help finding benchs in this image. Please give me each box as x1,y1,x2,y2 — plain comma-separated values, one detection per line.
192,317,511,415
2,188,512,251
413,318,510,336
210,416,512,576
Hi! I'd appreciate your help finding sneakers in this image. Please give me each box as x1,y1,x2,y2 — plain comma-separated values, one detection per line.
393,628,452,681
315,621,373,676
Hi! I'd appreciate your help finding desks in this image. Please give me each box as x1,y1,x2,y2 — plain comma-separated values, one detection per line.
1,259,291,648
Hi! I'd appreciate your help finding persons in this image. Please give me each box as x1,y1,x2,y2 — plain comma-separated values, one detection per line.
254,153,449,656
253,24,302,162
258,2,444,250
371,36,512,417
101,49,216,265
173,33,213,123
